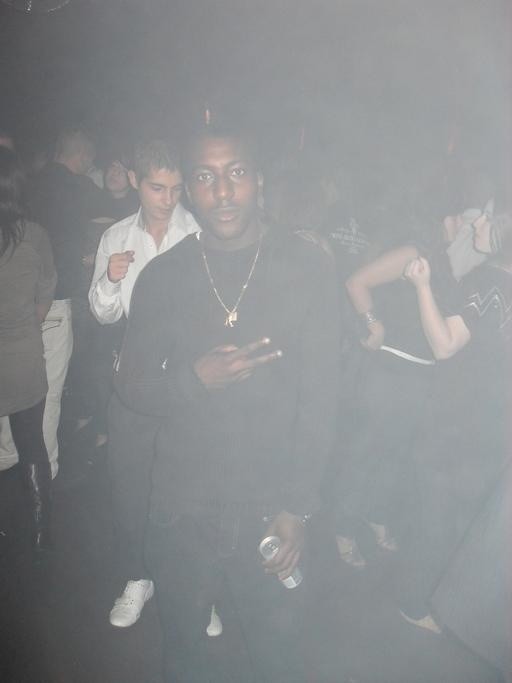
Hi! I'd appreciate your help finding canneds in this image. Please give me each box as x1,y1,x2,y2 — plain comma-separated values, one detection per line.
259,534,303,590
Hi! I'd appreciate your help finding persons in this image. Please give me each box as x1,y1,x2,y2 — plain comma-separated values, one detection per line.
1,120,511,682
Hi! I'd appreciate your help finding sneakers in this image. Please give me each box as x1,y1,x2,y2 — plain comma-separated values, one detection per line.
107,576,158,628
395,607,441,636
205,607,224,639
332,525,400,570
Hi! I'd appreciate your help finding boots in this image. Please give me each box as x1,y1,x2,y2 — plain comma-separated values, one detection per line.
19,459,55,557
